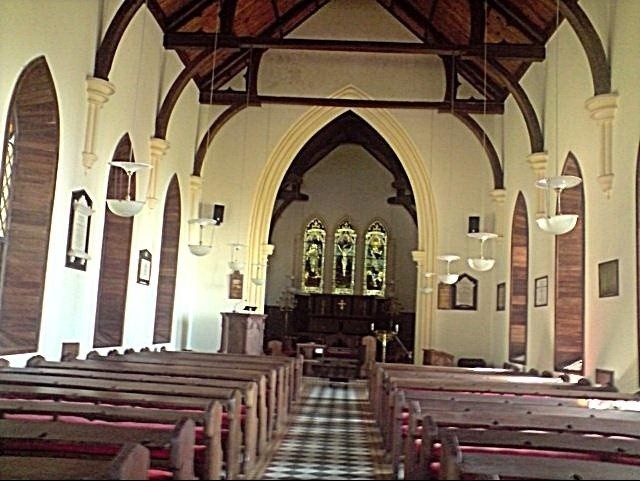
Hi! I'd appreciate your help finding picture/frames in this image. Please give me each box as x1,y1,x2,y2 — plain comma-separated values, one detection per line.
597,258,619,299
497,282,505,312
534,276,549,308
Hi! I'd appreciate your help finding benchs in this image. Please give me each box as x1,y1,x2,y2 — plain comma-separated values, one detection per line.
368,352,640,481
0,348,303,481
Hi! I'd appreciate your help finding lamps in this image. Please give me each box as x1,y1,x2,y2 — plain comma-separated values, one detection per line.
184,0,222,258
532,0,583,237
436,0,499,286
104,1,155,219
